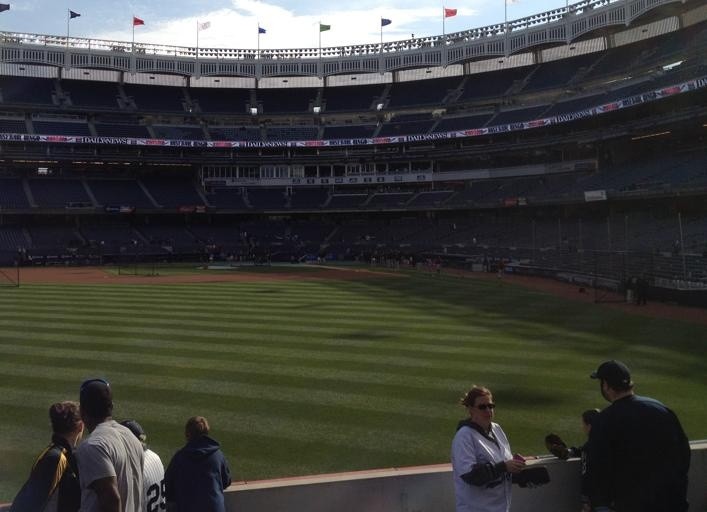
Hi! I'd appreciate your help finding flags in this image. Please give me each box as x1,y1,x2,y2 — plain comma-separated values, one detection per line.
0,3,9,12
258,27,266,34
69,10,81,19
445,8,456,18
319,23,330,32
132,16,144,26
197,21,209,30
381,18,391,27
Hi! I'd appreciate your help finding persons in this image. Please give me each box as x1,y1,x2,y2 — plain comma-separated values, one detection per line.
449,385,546,511
162,414,233,511
584,357,691,511
305,166,454,195
10,398,85,512
1,227,705,307
544,408,602,510
74,376,145,512
117,419,167,511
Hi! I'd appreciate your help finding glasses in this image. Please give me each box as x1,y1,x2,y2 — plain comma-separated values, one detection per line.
472,403,494,410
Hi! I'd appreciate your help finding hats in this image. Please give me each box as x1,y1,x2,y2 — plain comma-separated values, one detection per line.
78,378,112,411
118,418,146,438
589,358,630,386
